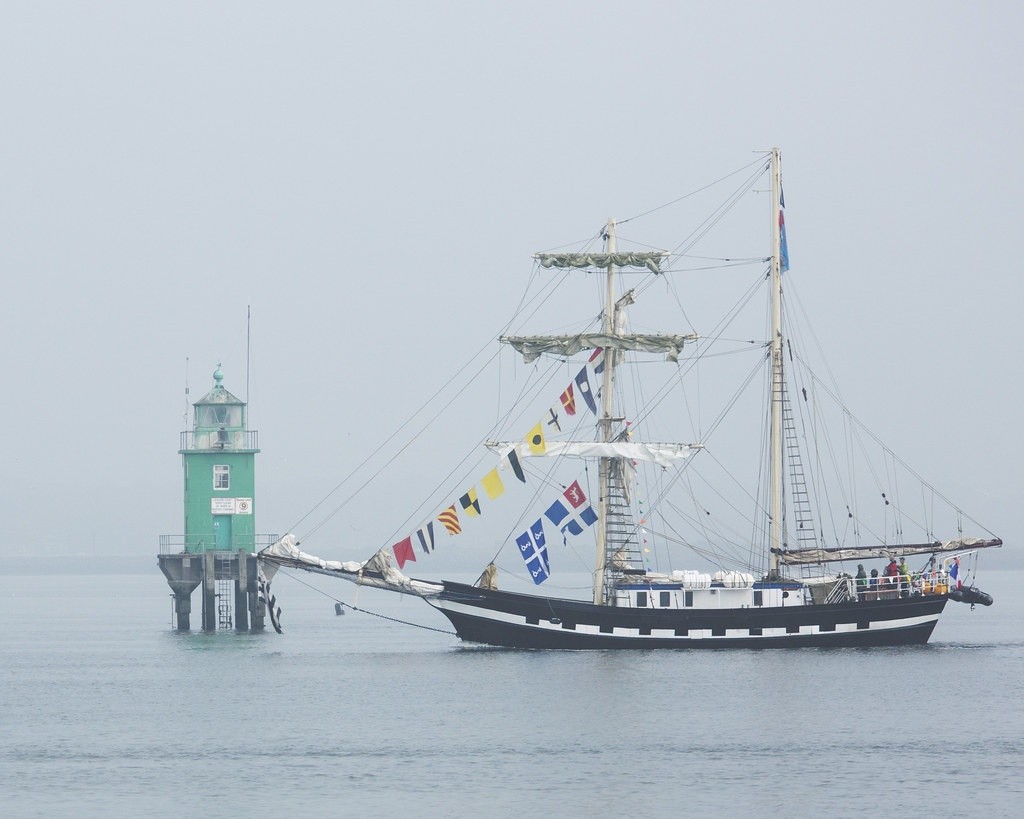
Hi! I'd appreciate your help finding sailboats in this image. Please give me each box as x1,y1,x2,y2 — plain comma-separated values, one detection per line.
248,147,1005,650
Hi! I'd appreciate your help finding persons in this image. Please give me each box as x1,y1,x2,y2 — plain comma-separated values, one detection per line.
870,569,880,587
887,557,898,589
856,564,867,589
897,557,945,592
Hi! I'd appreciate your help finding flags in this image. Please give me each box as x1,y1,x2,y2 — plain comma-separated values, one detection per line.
559,382,576,415
415,521,434,554
544,480,598,548
480,468,504,500
949,563,961,589
437,504,462,537
525,421,546,455
588,347,605,374
780,191,790,275
544,404,562,434
391,536,416,569
516,517,551,585
458,488,481,521
575,366,597,416
